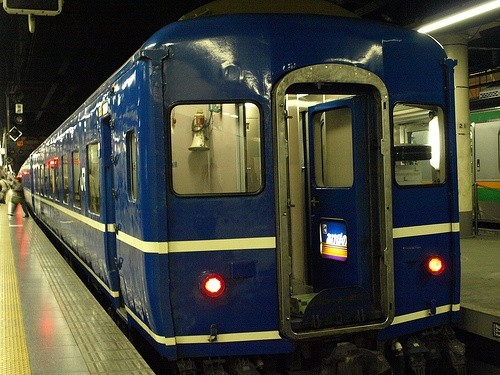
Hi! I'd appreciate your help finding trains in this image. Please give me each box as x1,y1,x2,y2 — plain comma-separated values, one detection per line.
15,3,460,365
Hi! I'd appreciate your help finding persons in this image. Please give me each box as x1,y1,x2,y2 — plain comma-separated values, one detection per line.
7,176,30,218
89,168,98,213
0,175,8,204
43,176,50,196
